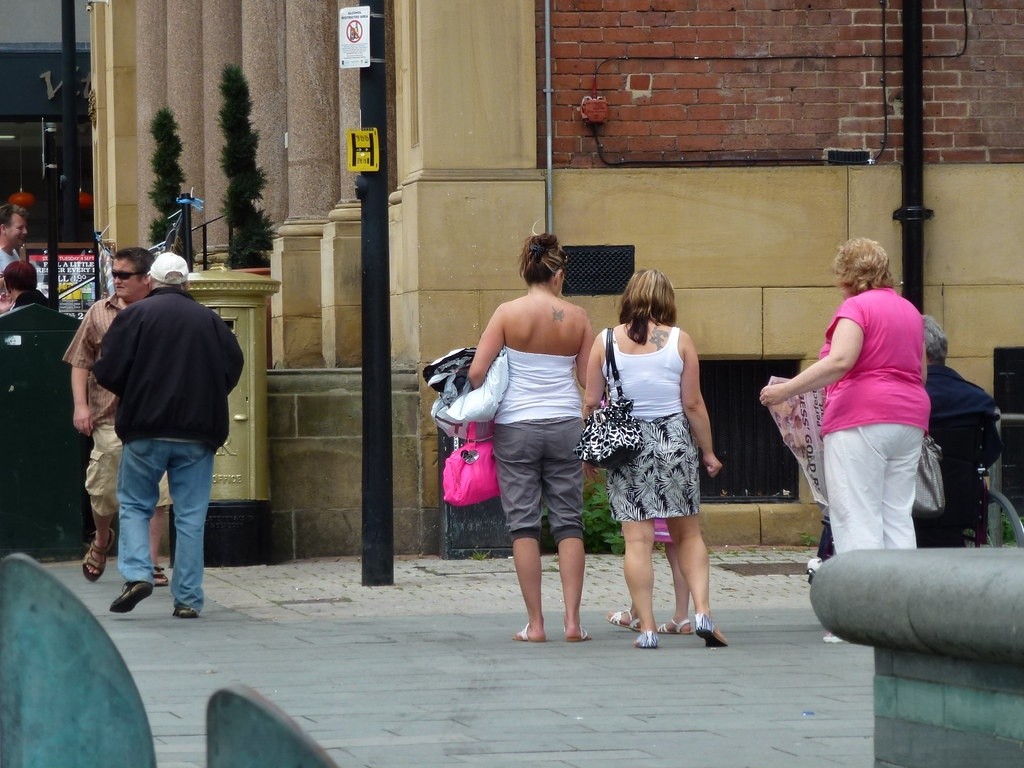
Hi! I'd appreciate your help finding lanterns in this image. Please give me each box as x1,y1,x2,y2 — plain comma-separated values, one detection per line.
11,192,35,207
80,193,93,206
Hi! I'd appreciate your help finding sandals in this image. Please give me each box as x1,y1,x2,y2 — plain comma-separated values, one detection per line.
606,606,642,631
82,527,116,582
656,616,694,634
153,564,168,584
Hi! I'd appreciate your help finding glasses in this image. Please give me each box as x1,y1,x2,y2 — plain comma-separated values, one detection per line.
111,269,148,280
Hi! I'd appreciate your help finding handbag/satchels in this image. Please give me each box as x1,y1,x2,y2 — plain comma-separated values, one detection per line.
442,422,500,506
573,328,645,469
911,436,946,516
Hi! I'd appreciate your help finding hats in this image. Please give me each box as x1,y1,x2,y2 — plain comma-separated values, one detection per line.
147,252,189,284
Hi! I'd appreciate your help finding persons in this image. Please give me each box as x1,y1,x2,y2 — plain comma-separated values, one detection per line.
0,204,28,292
470,234,595,642
0,261,48,314
584,269,730,648
91,252,245,618
758,237,999,643
61,248,170,585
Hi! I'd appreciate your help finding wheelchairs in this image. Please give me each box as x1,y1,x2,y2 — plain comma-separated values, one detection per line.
806,412,1024,585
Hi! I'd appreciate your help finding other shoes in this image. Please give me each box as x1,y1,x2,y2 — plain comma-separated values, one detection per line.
110,581,153,613
693,614,729,647
172,602,195,618
822,634,843,644
633,631,659,649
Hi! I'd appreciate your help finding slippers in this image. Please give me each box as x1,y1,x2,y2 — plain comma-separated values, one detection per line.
562,622,593,641
513,622,547,643
